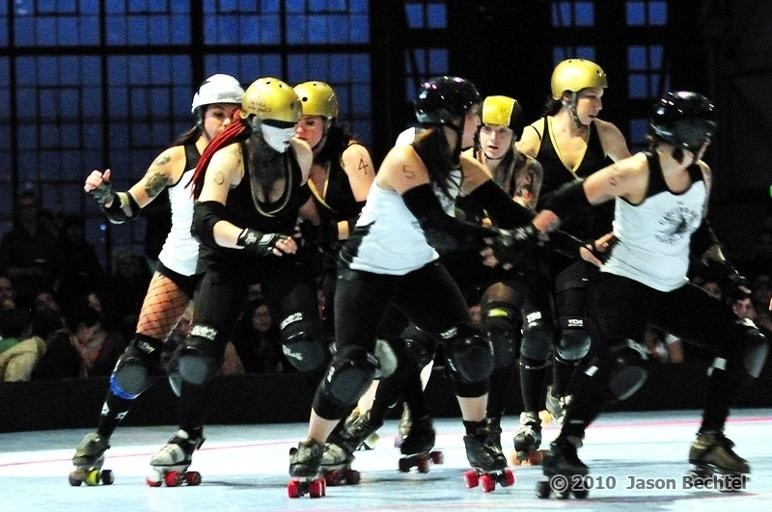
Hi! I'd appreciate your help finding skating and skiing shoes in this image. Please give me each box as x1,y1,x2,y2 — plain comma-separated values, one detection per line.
536,440,590,500
68,433,113,487
146,427,206,486
538,386,571,426
288,406,543,498
688,430,751,486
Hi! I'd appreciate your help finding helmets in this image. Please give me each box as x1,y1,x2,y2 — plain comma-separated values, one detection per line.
292,81,339,121
238,78,305,124
550,59,609,101
648,90,717,151
415,76,482,130
478,95,524,142
191,73,245,114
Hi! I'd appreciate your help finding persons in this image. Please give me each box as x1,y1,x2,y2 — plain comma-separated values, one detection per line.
1,53,772,482
